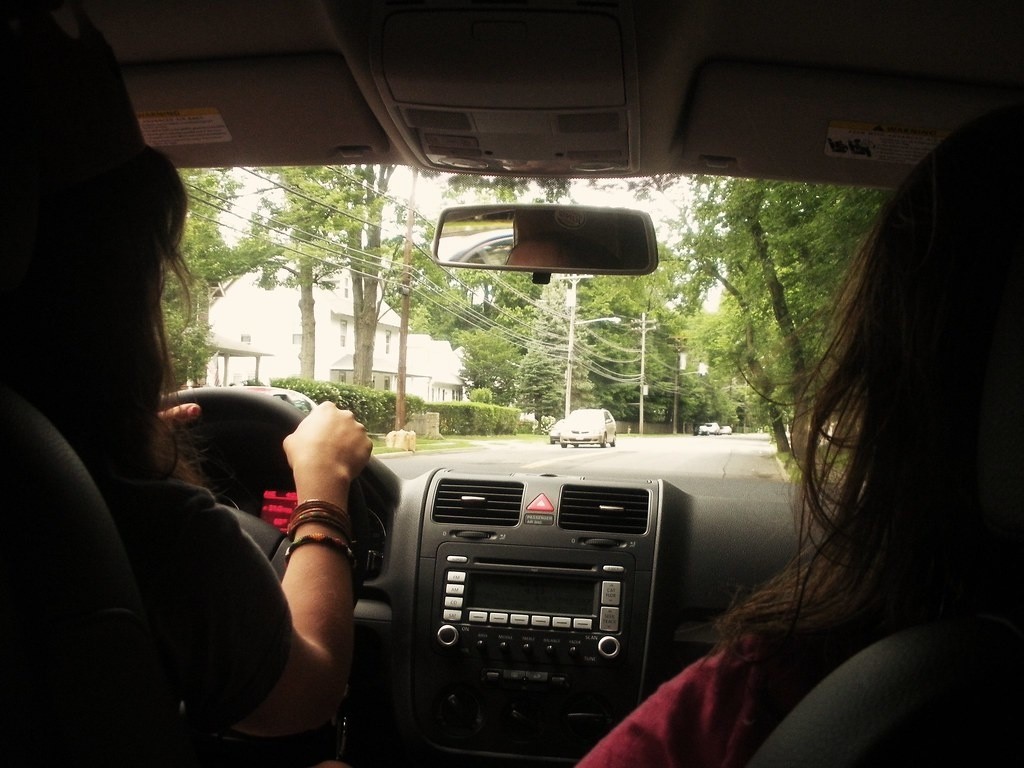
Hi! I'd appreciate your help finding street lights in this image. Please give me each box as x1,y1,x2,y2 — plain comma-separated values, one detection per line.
564,317,621,418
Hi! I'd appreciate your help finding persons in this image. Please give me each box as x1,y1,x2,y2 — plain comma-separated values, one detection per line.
0,1,376,768
507,231,622,269
573,108,1024,768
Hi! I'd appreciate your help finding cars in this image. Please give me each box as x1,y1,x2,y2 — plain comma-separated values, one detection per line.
549,419,565,445
560,408,617,449
699,423,733,435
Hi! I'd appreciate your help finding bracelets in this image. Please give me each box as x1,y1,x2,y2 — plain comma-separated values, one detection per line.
284,500,357,570
